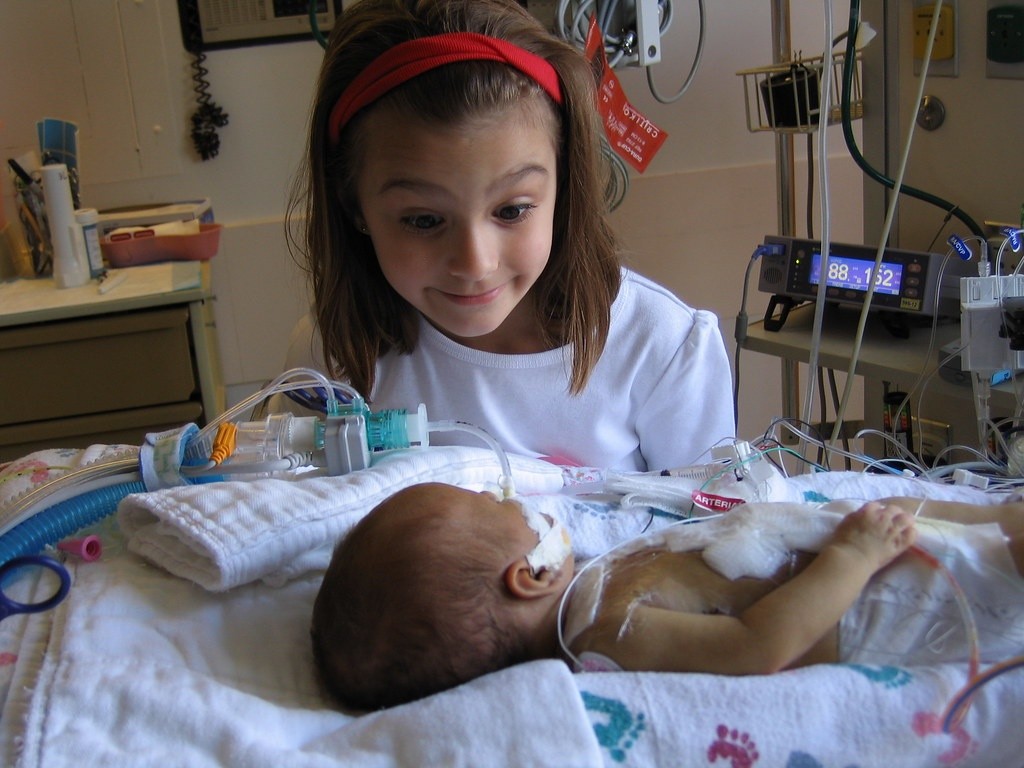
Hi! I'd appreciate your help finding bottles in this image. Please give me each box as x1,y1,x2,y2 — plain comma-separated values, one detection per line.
69,208,108,280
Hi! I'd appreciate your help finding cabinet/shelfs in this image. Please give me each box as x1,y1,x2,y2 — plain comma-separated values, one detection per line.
0,258,224,466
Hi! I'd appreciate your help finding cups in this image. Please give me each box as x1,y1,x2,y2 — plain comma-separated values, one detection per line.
0,175,82,279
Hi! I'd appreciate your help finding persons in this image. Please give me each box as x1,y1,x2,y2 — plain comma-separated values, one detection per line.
275,0,738,479
311,479,1024,715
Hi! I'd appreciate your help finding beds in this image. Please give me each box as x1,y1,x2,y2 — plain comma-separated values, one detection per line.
0,434,1024,768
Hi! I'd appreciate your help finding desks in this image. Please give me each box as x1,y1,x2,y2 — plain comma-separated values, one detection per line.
739,300,1024,477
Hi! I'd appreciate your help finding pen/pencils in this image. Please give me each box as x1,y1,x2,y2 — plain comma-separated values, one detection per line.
98,272,128,295
8,158,44,194
